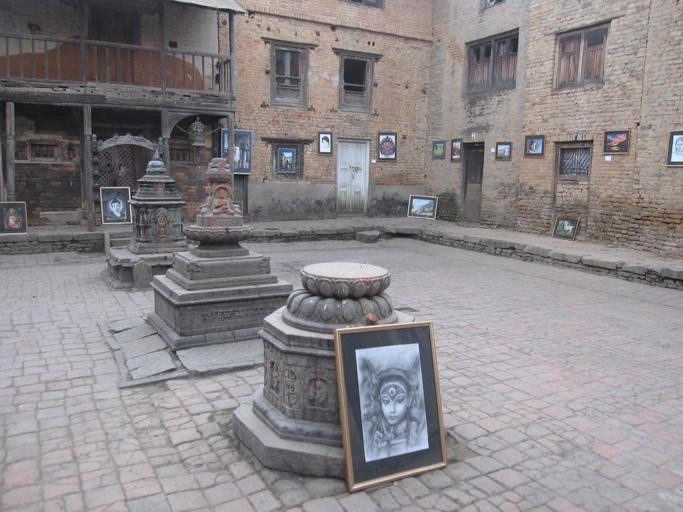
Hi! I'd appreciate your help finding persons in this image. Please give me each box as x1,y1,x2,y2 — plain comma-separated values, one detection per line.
369,368,426,459
322,136,329,146
527,141,536,153
673,137,683,156
108,198,125,219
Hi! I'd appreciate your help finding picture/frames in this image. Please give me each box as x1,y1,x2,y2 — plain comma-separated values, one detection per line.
316,131,332,154
602,129,631,156
375,131,397,162
332,319,448,494
0,199,28,236
450,138,463,162
495,141,512,161
552,216,580,241
665,130,683,167
220,127,253,176
523,135,545,158
406,194,438,220
99,185,132,226
276,146,297,174
431,140,445,159
